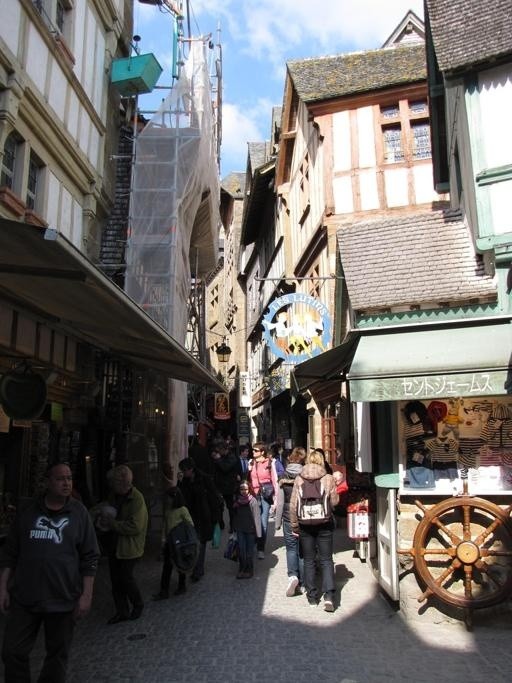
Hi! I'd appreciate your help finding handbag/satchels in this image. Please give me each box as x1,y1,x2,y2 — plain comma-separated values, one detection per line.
258,483,274,500
207,488,225,511
223,535,239,561
212,520,220,549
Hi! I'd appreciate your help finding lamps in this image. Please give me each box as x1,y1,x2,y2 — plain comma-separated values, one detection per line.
215,336,232,362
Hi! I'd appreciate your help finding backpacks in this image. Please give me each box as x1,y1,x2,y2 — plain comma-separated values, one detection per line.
296,473,333,527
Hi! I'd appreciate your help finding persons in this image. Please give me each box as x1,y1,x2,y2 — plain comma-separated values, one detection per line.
150,435,340,613
1,463,102,683
87,464,148,625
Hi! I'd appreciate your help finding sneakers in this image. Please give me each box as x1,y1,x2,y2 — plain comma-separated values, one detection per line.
190,570,206,582
108,611,130,624
128,603,143,620
228,526,238,533
257,551,265,559
324,592,335,612
174,587,185,594
307,598,318,607
236,570,254,579
152,591,169,599
285,575,306,596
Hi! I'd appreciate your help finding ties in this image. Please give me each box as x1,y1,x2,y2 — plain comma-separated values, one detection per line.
242,459,247,473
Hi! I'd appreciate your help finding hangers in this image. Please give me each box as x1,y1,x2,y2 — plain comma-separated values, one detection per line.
437,431,449,439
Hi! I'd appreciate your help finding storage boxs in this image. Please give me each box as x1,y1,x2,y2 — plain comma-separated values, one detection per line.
346,512,368,539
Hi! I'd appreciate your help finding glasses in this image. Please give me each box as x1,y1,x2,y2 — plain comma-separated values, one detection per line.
252,448,261,452
